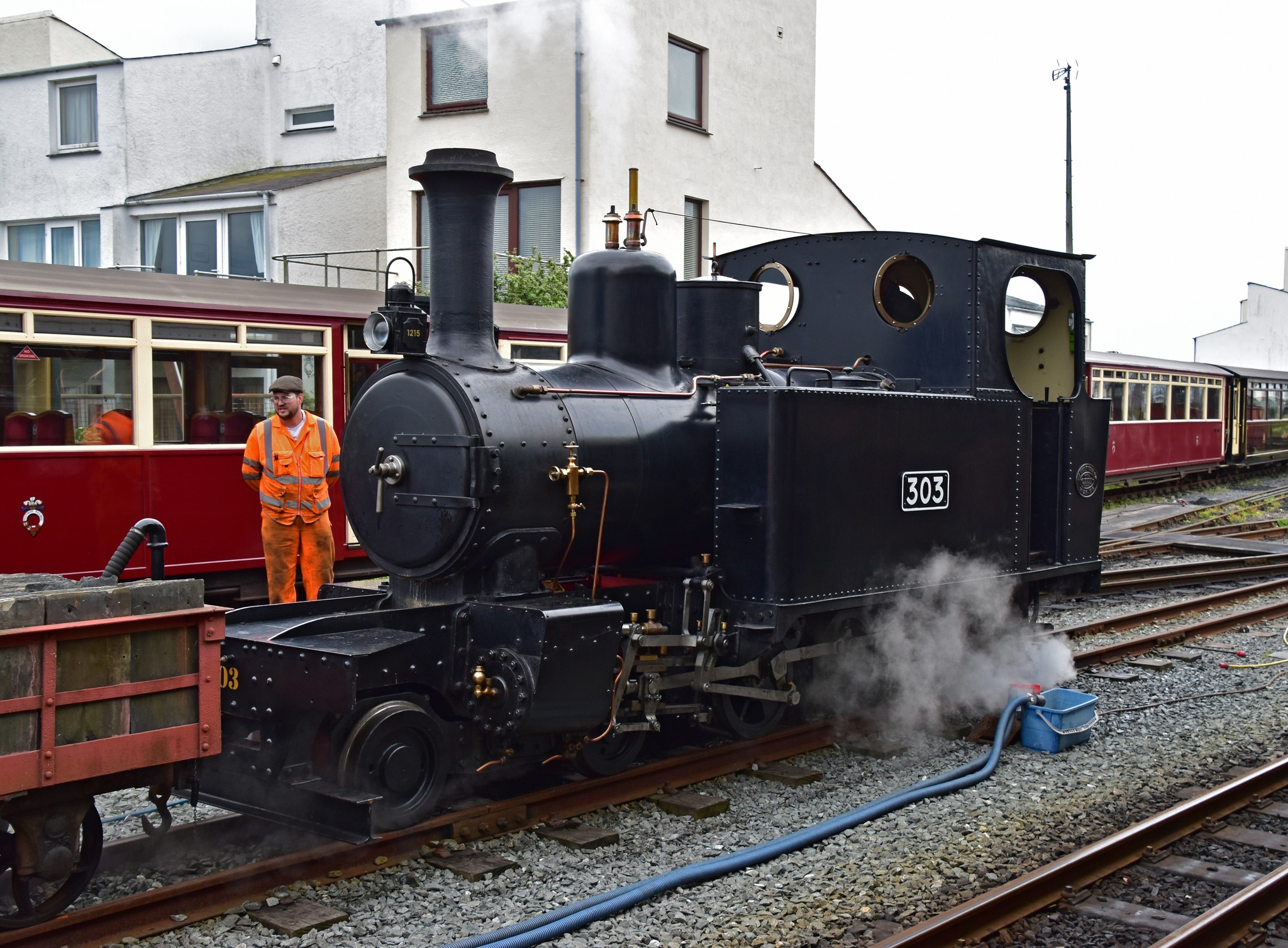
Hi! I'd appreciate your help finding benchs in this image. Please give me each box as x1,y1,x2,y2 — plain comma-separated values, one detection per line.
191,412,254,444
4,410,77,446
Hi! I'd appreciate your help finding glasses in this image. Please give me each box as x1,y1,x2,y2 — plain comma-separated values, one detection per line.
270,394,298,405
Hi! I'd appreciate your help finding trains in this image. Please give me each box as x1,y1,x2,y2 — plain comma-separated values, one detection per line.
1,257,1288,583
1,146,1118,932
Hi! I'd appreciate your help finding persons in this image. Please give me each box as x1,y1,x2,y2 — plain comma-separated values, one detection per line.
82,409,134,445
241,375,341,605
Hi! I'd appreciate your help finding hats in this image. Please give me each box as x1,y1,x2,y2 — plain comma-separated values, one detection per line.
268,375,303,393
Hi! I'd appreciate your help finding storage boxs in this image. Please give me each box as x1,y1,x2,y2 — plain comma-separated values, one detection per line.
1020,687,1099,755
